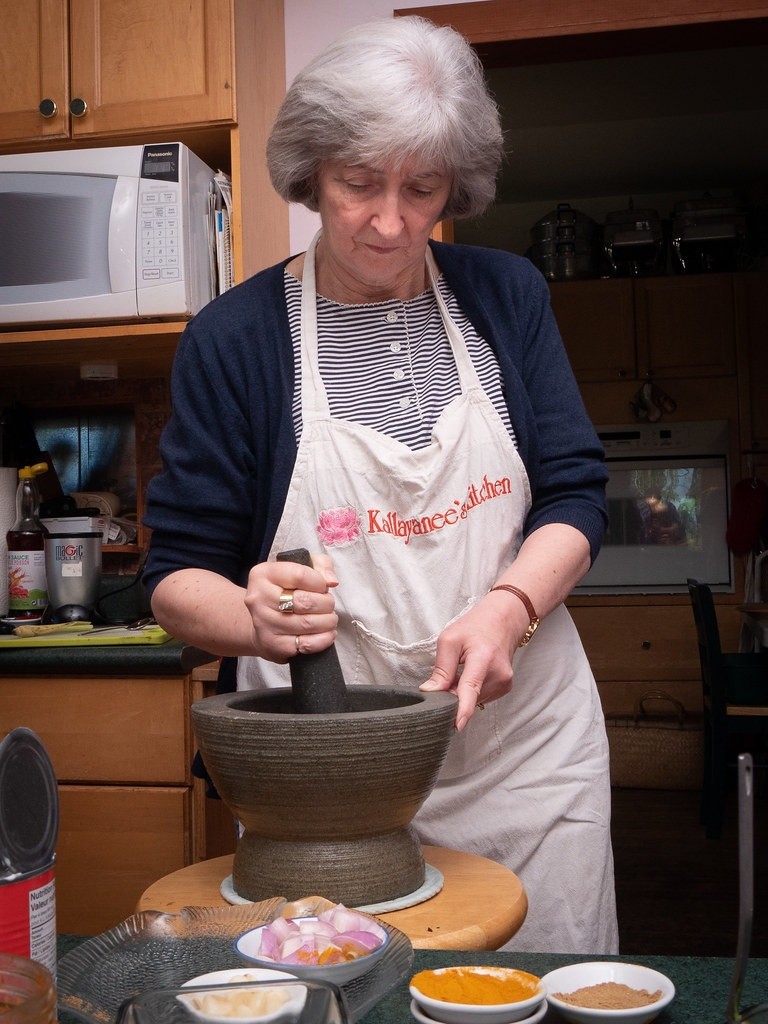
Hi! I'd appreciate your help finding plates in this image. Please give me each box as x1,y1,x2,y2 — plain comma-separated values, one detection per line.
57,896,414,1024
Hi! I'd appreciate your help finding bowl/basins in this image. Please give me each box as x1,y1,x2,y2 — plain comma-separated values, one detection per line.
542,961,675,1024
410,998,548,1024
234,916,390,984
173,968,308,1022
409,966,546,1024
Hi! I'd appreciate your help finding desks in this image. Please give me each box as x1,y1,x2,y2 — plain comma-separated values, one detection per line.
1,642,222,938
55,932,768,1023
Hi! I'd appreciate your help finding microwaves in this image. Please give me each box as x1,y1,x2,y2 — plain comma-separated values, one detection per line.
1,141,215,325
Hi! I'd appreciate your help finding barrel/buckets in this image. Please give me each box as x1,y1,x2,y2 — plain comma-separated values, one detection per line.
0,725,57,992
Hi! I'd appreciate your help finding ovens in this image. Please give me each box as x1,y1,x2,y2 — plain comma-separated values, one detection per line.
573,420,729,586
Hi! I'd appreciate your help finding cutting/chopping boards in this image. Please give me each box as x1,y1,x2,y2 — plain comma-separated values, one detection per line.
0,625,171,647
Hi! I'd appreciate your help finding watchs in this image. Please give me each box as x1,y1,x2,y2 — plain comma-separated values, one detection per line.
489,585,540,648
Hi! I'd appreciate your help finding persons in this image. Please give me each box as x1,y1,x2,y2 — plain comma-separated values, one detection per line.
139,15,619,957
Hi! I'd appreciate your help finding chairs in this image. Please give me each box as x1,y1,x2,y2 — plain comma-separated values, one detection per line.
686,578,768,1024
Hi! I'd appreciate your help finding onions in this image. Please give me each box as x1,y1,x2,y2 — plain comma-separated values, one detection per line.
259,901,382,967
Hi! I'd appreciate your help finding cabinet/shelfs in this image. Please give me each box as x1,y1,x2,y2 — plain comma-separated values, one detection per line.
530,273,767,737
0,0,287,137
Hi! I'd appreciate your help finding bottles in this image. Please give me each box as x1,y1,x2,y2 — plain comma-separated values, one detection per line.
6,462,49,619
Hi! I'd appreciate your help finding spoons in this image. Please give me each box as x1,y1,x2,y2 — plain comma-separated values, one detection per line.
77,618,153,636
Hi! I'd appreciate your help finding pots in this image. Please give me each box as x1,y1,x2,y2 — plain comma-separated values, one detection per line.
528,191,748,282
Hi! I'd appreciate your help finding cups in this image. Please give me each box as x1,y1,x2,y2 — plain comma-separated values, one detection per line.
44,532,104,613
0,952,57,1023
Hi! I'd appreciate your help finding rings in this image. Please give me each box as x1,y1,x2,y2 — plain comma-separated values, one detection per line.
295,635,300,654
477,703,484,711
277,588,295,614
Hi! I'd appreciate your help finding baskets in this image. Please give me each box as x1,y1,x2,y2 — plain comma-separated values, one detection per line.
606,689,710,791
